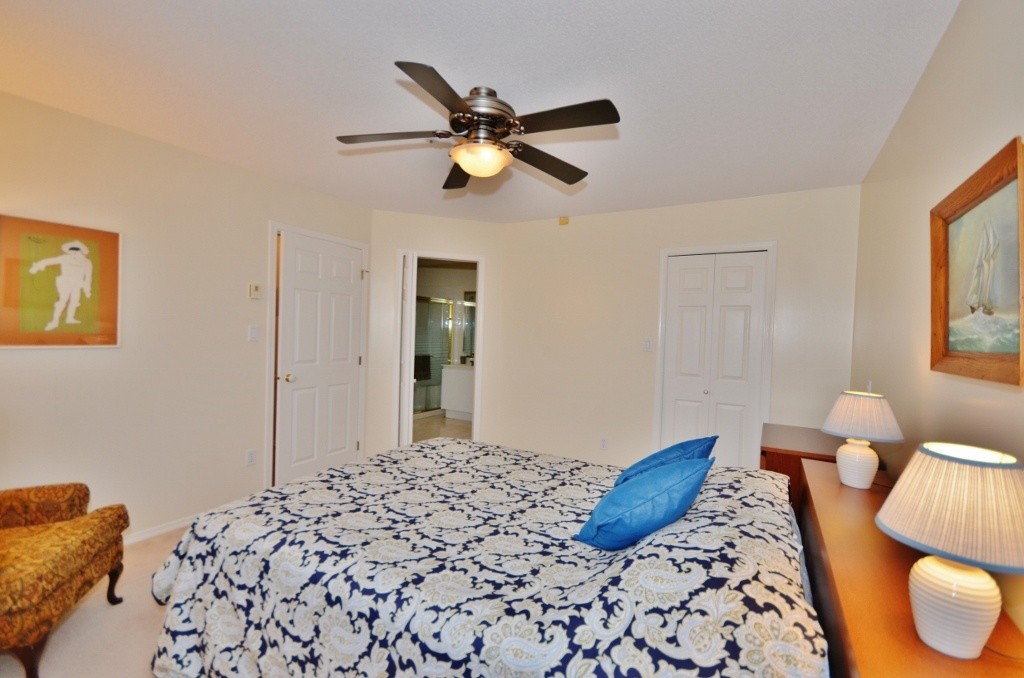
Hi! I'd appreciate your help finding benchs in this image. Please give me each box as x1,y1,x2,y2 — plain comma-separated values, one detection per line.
0,482,132,678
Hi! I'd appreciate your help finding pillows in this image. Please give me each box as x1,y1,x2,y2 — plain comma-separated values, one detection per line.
572,455,717,550
614,435,720,488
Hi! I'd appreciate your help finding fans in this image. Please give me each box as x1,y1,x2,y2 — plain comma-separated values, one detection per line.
335,61,621,189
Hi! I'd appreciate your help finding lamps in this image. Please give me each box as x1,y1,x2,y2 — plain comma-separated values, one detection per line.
820,390,905,490
875,441,1024,660
448,139,515,178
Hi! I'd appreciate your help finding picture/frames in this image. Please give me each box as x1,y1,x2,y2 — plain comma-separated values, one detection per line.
930,135,1024,387
0,213,122,350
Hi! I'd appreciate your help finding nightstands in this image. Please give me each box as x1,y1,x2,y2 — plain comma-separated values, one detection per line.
759,422,883,514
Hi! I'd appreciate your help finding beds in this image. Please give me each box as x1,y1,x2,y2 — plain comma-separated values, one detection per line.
148,435,1024,678
